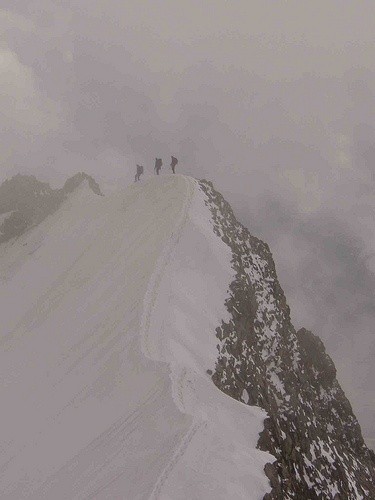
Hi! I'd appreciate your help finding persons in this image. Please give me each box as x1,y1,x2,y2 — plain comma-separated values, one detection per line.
170,155,177,172
154,158,160,175
134,164,142,181
